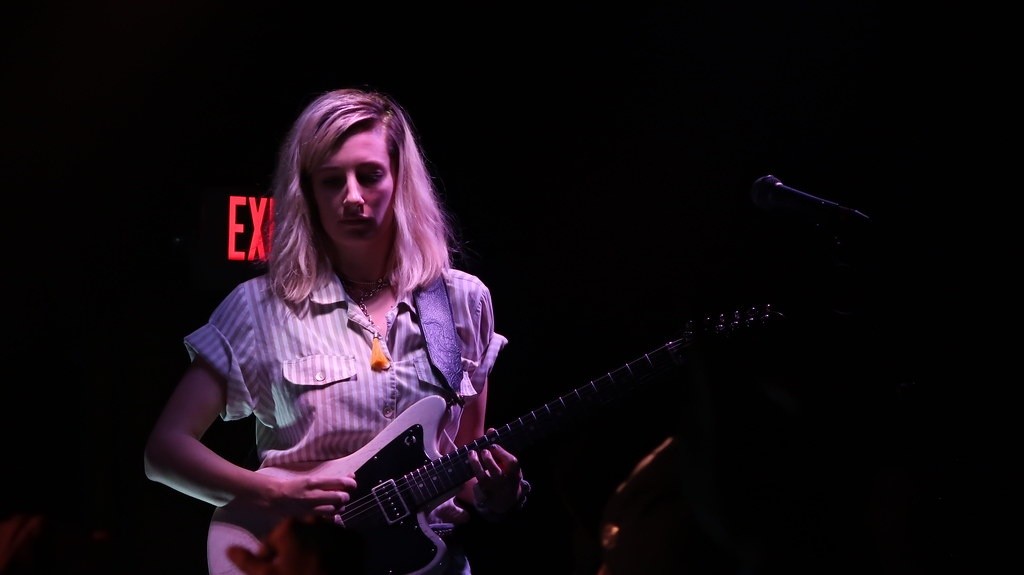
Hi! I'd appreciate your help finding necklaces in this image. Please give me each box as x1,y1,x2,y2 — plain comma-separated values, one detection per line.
340,276,390,371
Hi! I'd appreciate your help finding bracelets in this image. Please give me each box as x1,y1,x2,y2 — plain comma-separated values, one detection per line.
516,479,532,512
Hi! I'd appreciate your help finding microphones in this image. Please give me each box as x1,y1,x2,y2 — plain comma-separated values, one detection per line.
752,175,870,221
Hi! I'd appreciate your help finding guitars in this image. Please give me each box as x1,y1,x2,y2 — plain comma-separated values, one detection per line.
206,301,788,575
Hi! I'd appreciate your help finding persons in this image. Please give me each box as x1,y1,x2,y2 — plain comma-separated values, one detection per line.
145,88,531,575
